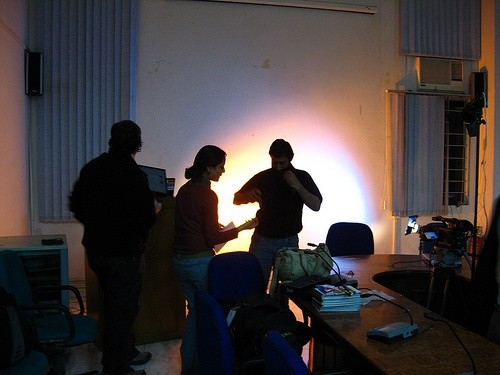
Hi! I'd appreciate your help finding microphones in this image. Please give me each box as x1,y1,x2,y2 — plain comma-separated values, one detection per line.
304,243,358,290
361,293,418,344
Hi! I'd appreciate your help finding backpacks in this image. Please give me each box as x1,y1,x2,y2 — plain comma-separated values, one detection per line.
229,299,303,375
0,287,33,370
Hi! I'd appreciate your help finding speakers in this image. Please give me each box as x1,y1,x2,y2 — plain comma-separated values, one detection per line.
471,72,487,107
25,52,43,96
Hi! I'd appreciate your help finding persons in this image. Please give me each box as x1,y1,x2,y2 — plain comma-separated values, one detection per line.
68,120,157,375
233,139,323,309
172,145,259,312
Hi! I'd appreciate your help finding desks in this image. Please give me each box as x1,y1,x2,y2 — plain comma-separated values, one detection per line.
280,254,500,375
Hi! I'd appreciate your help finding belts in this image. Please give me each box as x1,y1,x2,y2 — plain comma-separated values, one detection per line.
175,251,212,259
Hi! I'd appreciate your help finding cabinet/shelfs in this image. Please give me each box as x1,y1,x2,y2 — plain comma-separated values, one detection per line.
84,198,187,351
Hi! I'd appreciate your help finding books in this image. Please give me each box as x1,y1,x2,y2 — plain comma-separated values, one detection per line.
311,284,361,312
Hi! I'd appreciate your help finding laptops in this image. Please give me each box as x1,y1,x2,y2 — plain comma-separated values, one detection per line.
137,165,168,206
161,178,175,198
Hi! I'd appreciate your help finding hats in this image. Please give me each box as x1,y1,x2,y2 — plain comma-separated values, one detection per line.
111,120,141,138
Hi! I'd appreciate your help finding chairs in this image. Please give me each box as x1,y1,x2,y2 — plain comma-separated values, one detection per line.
191,222,375,375
0,250,101,375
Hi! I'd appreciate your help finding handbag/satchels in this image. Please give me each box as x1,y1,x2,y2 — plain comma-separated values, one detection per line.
272,243,334,281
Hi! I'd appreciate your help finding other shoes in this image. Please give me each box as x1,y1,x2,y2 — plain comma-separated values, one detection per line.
102,368,146,375
130,351,152,365
